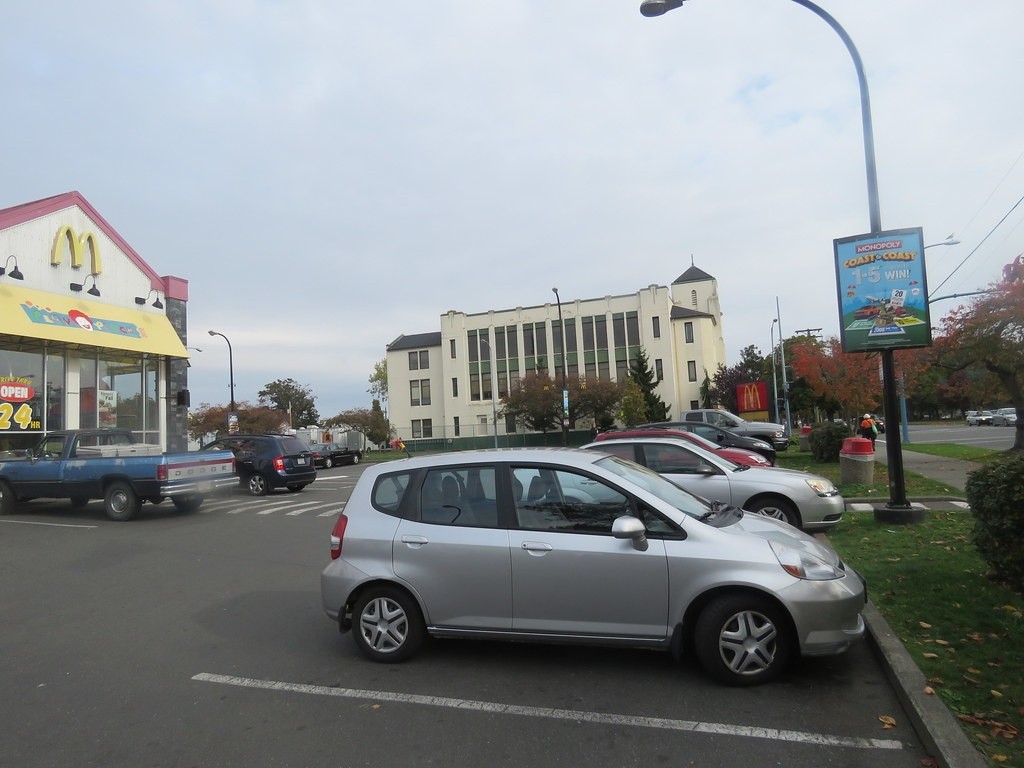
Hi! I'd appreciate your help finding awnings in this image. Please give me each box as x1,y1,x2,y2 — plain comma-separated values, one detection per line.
0,284,189,360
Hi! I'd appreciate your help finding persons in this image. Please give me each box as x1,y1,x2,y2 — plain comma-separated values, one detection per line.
861,414,877,452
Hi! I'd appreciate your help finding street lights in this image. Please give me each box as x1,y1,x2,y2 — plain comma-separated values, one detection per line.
551,286,571,447
637,0,926,526
480,339,499,449
890,237,962,446
770,318,782,425
207,329,238,436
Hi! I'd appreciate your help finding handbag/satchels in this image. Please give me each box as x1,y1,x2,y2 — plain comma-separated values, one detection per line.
871,420,878,434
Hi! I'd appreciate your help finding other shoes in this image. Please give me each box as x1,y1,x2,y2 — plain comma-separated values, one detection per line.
873,447,875,451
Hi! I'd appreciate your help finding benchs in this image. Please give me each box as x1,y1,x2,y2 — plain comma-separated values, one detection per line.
429,474,478,524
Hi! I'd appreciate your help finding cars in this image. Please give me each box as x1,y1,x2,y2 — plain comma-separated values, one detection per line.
317,445,869,689
512,437,846,535
992,407,1017,427
309,442,363,469
965,409,997,427
591,426,773,472
824,418,848,426
636,420,777,467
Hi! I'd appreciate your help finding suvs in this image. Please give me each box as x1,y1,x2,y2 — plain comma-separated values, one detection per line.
196,432,317,497
856,414,885,435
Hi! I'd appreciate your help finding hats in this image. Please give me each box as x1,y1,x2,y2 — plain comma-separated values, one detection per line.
864,414,870,419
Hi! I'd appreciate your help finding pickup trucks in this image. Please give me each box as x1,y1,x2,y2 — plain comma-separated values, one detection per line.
663,408,791,451
0,427,241,522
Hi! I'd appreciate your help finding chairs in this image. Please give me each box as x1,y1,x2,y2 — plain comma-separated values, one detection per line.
514,476,556,530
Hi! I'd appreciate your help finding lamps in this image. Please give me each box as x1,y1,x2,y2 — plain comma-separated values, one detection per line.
169,389,190,409
135,288,163,309
70,274,100,296
0,255,24,280
